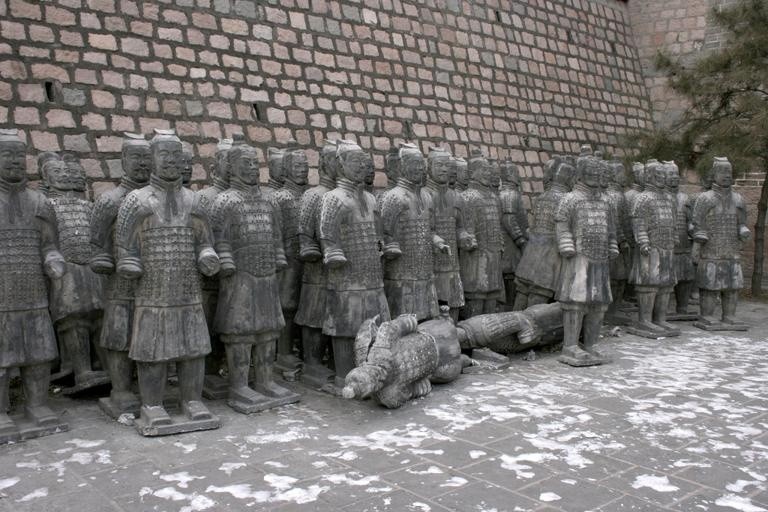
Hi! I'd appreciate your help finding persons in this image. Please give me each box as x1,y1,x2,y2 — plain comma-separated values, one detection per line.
511,149,751,366
0,130,548,436
